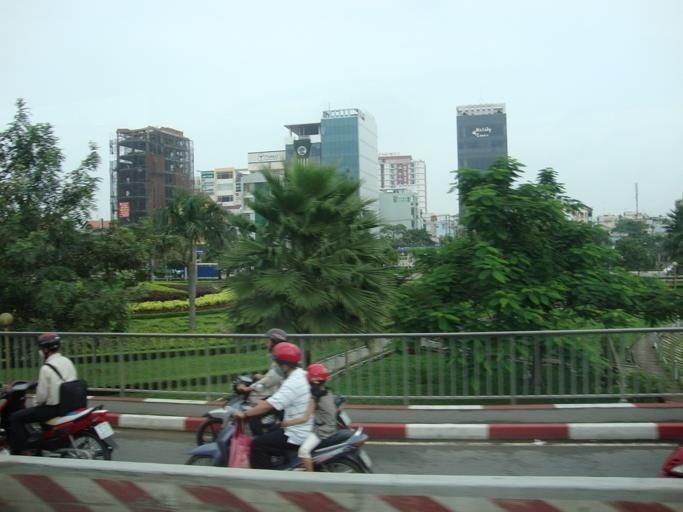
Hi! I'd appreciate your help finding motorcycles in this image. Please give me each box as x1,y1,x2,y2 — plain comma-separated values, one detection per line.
0,380,118,460
191,375,352,445
186,403,375,473
656,444,682,479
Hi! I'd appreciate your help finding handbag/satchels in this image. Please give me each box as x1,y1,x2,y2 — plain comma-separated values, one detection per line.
228,418,251,468
60,378,87,413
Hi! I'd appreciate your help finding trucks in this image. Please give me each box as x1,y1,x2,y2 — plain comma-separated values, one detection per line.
178,263,219,279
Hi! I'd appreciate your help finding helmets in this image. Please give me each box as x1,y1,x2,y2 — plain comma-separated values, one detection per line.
272,341,301,363
305,363,327,384
267,328,288,344
36,332,61,352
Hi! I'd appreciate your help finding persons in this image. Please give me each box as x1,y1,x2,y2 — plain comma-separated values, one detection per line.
4,331,90,458
278,361,343,473
237,328,290,426
229,342,316,466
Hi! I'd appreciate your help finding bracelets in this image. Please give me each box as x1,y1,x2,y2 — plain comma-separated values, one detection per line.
241,411,249,419
